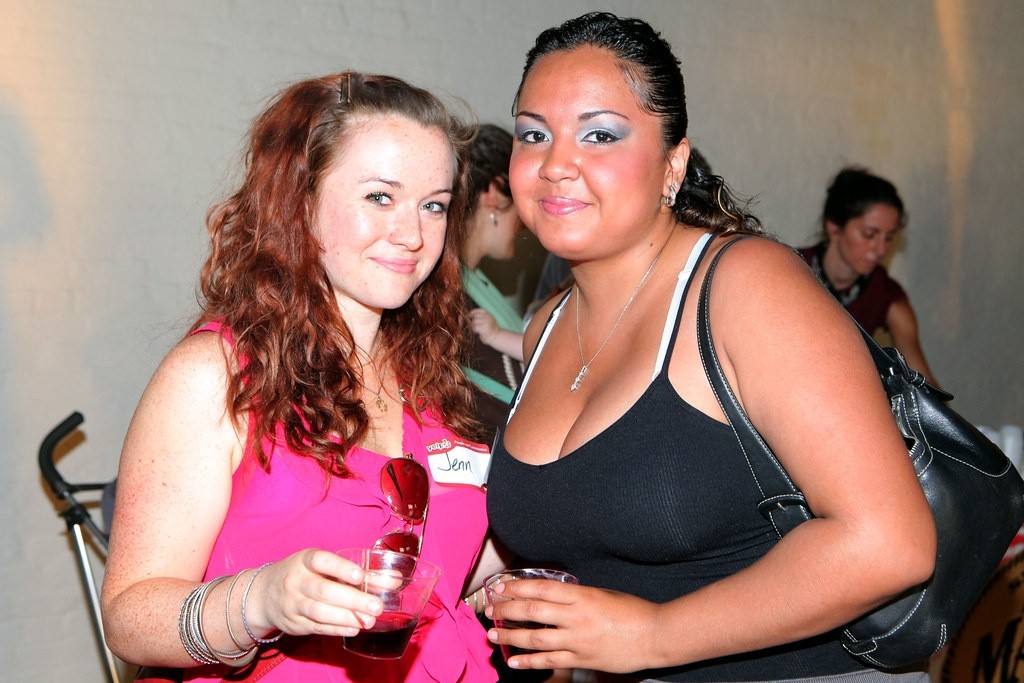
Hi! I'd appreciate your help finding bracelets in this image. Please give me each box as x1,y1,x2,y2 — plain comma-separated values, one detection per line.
473,592,478,615
479,587,486,620
226,568,257,650
242,562,284,643
464,597,470,607
179,575,259,667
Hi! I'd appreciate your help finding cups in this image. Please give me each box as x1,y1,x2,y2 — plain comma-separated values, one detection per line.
482,568,577,663
338,548,441,660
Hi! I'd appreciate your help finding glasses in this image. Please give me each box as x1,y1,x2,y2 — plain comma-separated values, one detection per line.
369,453,430,591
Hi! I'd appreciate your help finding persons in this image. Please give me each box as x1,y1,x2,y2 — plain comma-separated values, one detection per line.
797,166,939,387
462,125,525,445
487,9,941,683
101,71,493,683
468,309,523,362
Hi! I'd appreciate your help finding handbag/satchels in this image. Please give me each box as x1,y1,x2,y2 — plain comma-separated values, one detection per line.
696,232,1024,674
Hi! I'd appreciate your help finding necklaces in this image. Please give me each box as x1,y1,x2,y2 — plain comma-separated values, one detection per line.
359,344,389,412
569,250,662,393
